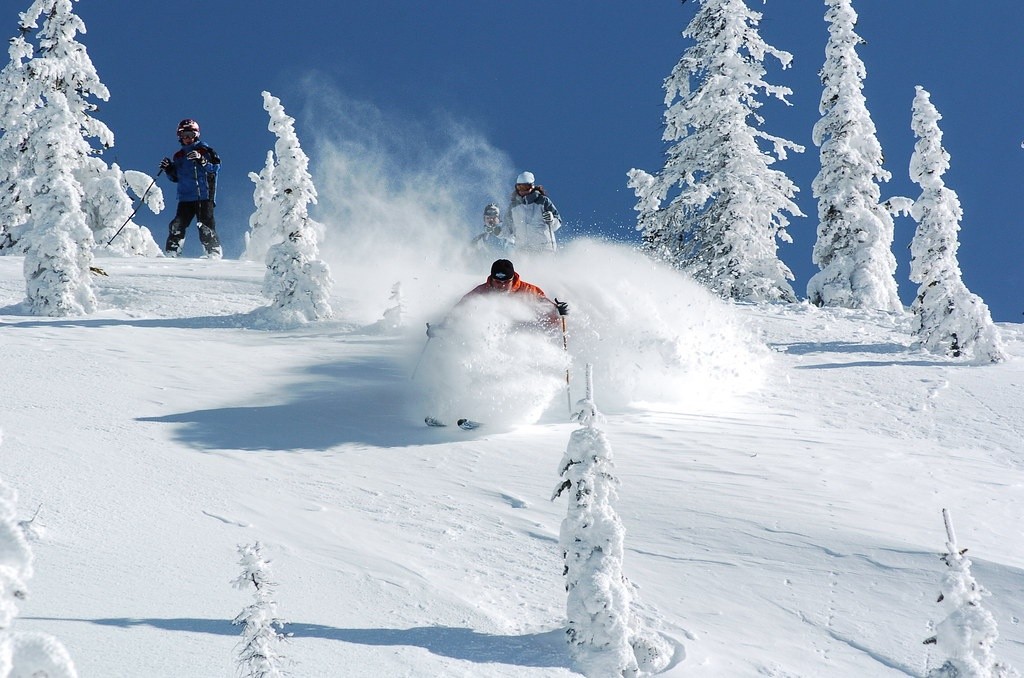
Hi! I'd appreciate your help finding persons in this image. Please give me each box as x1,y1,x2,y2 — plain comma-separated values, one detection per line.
494,171,562,252
158,119,223,259
469,203,514,258
426,259,570,426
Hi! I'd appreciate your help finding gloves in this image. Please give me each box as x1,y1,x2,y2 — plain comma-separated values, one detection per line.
159,157,171,172
543,211,553,223
186,150,201,161
494,225,501,236
426,322,435,338
555,297,569,315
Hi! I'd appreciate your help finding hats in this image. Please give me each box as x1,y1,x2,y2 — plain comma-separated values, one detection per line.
491,259,514,281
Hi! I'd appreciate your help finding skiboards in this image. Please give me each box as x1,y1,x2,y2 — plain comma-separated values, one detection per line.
425,416,480,430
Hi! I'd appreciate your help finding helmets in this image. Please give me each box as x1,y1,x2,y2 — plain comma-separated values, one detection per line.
484,203,501,220
517,172,535,196
177,119,200,138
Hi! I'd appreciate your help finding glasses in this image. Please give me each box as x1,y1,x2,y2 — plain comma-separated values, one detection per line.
515,182,533,191
179,131,196,139
